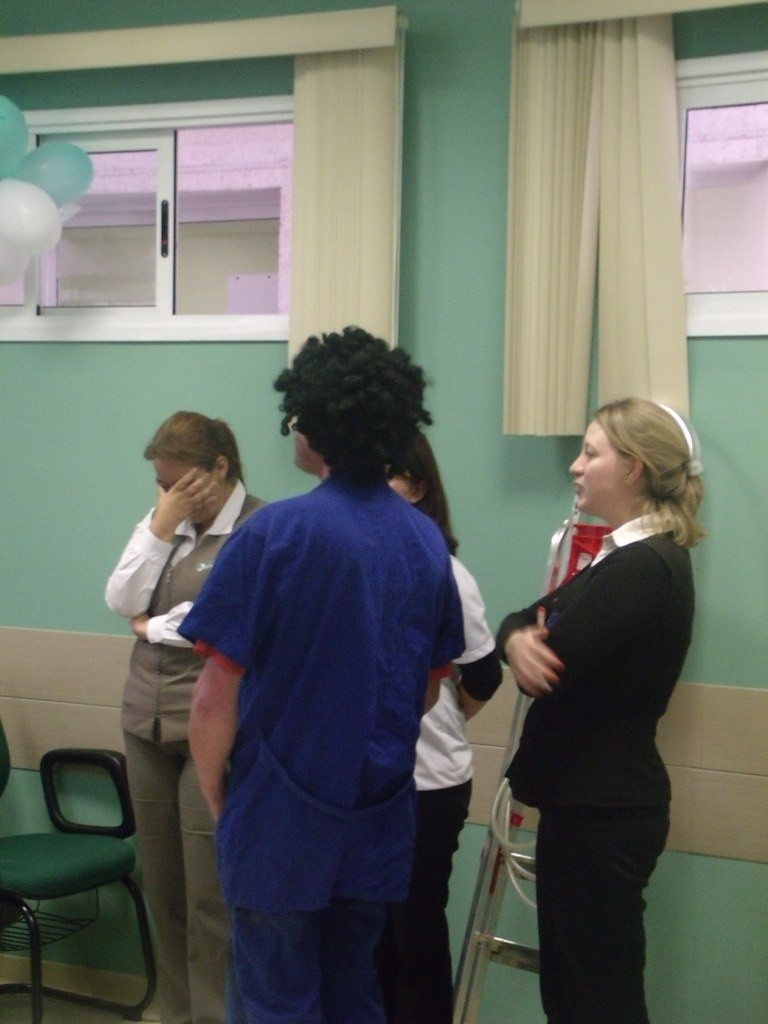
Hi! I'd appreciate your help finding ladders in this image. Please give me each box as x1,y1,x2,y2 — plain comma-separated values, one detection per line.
453,401,700,1024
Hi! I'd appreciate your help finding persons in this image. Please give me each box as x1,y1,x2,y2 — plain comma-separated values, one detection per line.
494,397,708,1024
176,323,505,1024
104,409,273,1024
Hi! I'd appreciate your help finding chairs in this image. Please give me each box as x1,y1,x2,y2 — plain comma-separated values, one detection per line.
0,722,158,1024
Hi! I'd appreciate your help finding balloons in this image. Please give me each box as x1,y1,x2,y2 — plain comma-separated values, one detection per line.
0,95,94,287
